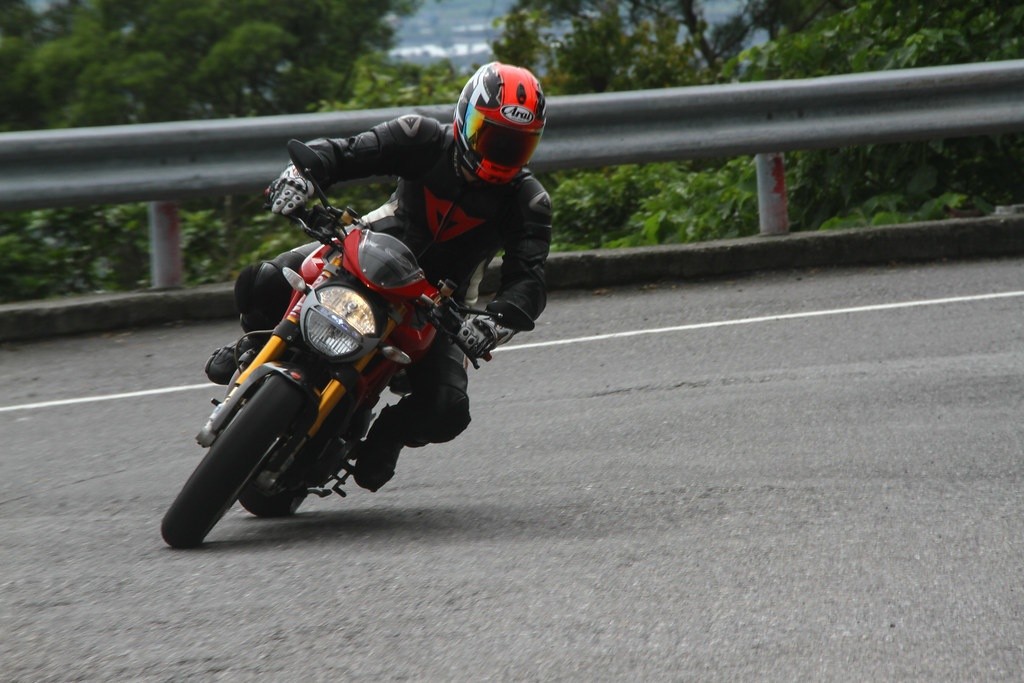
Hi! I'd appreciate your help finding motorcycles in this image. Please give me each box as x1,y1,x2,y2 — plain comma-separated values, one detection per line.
161,136,536,549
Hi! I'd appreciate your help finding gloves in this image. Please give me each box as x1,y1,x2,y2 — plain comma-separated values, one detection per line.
268,160,315,216
456,310,517,357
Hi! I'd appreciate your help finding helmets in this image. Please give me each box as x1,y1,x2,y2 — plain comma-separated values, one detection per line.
453,61,545,192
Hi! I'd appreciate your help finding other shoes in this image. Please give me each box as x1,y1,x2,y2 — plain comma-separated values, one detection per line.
355,403,403,492
205,337,267,385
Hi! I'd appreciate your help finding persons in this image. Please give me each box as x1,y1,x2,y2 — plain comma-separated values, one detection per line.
205,63,553,491
